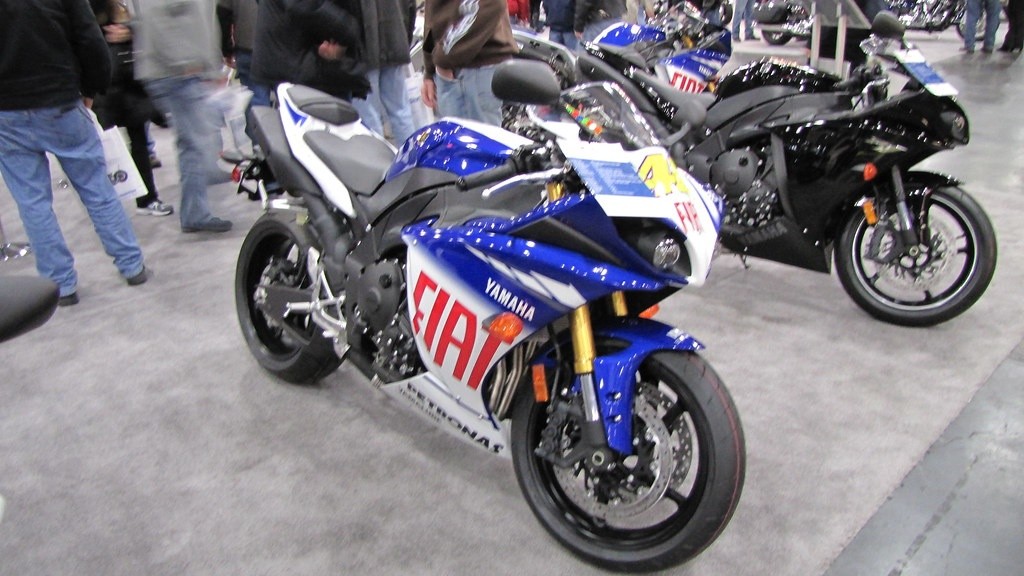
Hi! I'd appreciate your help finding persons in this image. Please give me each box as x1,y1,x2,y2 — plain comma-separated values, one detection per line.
1,0,149,306
90,0,1023,232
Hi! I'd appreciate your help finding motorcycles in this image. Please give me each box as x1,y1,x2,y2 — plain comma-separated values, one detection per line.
498,10,996,329
885,0,998,43
217,59,747,575
753,0,815,45
